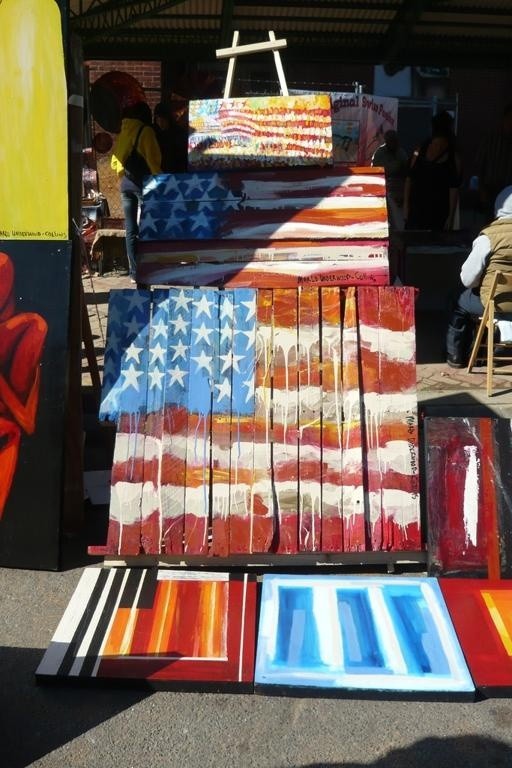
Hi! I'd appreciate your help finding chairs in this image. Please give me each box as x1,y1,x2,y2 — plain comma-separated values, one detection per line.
467,270,512,398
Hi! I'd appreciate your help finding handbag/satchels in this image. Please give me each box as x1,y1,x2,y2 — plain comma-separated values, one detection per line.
125,146,151,188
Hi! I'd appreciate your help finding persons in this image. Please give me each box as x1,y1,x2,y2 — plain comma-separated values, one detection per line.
443,184,512,370
151,102,189,174
371,128,411,234
400,108,463,232
108,99,166,284
